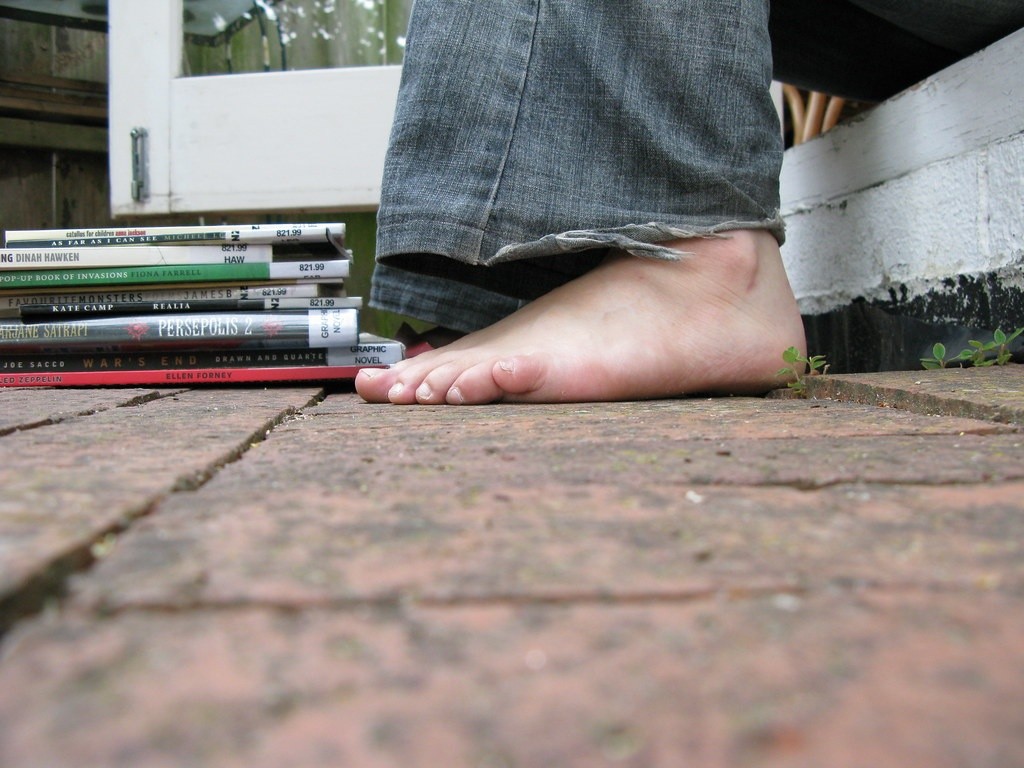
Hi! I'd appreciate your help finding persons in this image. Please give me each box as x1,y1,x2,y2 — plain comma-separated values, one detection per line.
354,0,1024,406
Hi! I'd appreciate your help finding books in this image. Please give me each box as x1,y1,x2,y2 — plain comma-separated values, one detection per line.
0,222,435,387
